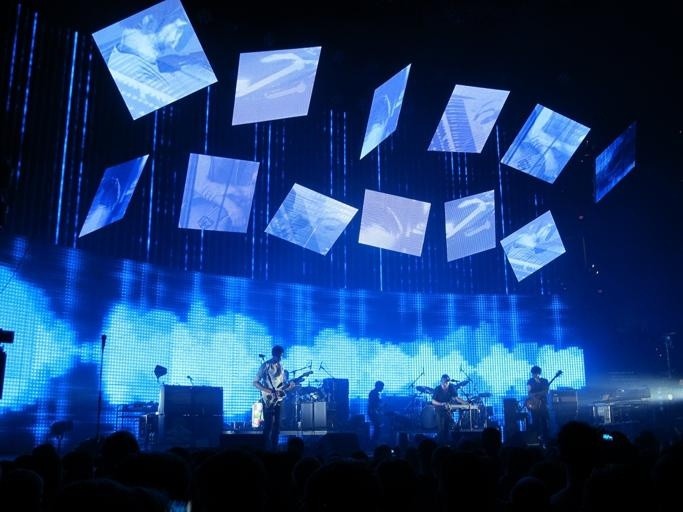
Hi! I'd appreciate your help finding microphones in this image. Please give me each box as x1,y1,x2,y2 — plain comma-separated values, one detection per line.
100,335,106,350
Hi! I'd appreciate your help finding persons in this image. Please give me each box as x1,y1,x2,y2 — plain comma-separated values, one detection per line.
280,370,293,418
431,374,464,434
368,381,384,441
527,365,549,435
107,0,217,115
360,64,411,160
253,343,295,453
78,158,138,238
1,419,683,511
194,155,259,235
518,106,590,184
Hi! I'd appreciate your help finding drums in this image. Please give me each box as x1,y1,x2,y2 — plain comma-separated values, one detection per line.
421,406,438,429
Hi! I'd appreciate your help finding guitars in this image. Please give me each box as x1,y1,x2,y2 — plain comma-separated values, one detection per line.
266,370,313,404
526,370,562,410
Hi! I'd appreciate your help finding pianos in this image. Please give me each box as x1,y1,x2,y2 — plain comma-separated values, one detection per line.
440,404,480,410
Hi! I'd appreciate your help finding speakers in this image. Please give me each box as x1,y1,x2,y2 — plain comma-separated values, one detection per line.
513,431,538,443
312,433,358,462
220,431,264,450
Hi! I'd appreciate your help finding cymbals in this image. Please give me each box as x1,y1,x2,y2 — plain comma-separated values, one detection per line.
416,386,434,394
